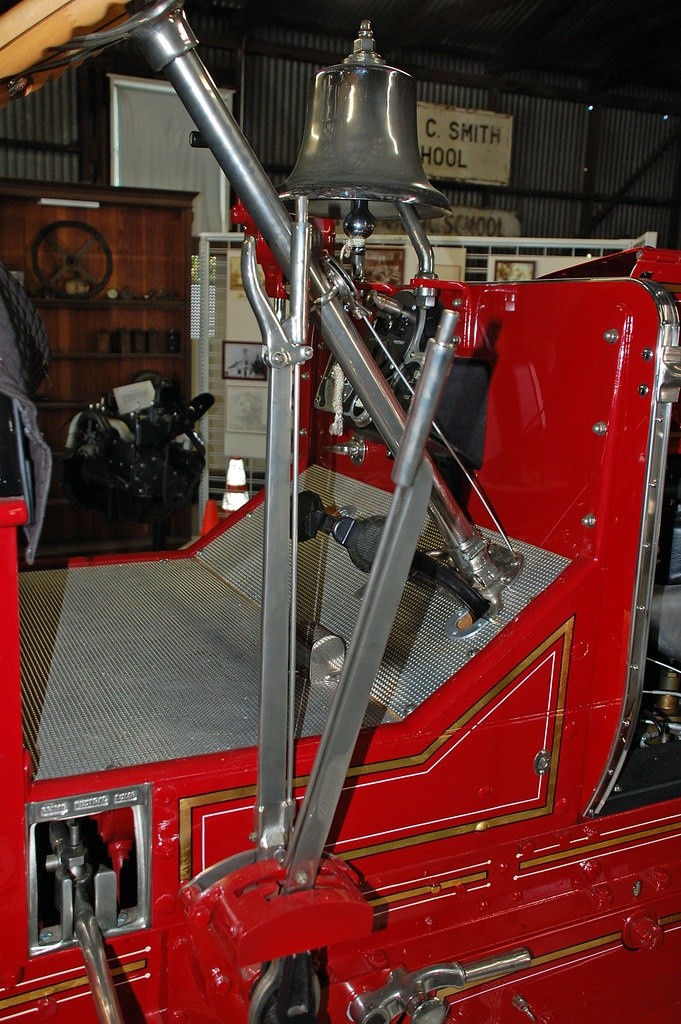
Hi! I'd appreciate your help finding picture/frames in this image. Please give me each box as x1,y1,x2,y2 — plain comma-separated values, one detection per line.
365,247,406,284
222,340,267,381
494,260,537,281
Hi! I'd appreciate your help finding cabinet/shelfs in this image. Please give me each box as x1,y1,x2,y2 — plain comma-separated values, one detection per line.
0,177,200,561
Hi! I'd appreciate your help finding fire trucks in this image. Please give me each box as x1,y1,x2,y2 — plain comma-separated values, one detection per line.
1,3,681,1024
